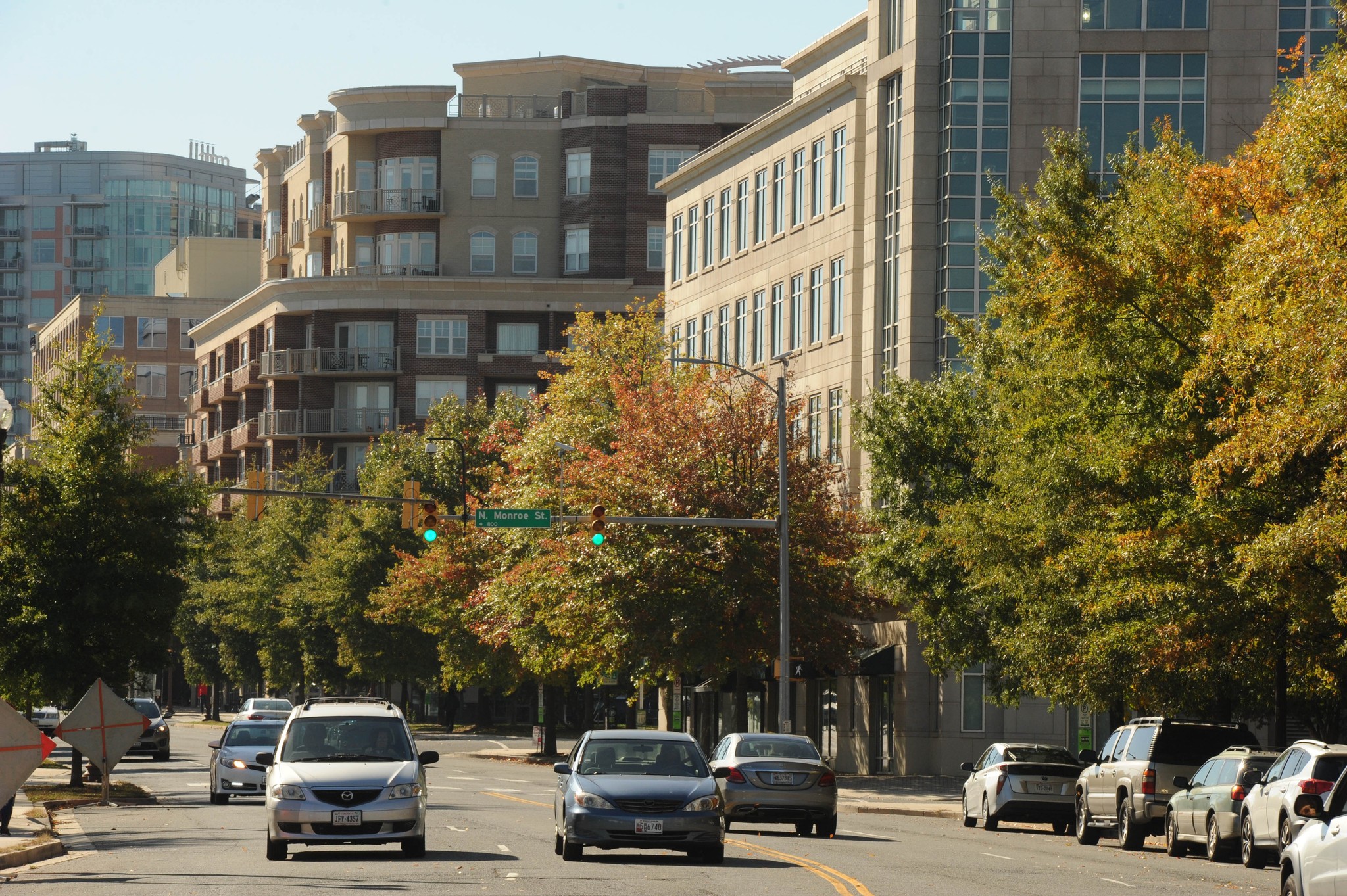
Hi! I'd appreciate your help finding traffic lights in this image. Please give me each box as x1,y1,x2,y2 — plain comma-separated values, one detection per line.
772,659,780,681
789,660,811,679
590,503,606,547
423,500,438,543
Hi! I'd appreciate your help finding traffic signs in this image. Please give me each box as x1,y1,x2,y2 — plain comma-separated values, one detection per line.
673,694,681,711
538,693,543,707
1077,702,1091,729
597,670,618,685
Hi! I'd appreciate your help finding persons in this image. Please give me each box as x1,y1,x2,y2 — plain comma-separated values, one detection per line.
648,744,691,776
359,728,402,760
197,681,207,714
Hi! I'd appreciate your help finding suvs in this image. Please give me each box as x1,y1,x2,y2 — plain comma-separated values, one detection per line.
253,695,440,858
1074,716,1263,852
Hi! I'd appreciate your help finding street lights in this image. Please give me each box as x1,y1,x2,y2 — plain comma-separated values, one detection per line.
664,358,790,734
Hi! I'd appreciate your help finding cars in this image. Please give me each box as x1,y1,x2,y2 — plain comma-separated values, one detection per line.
207,719,306,805
1279,764,1347,896
960,743,1085,835
1164,745,1285,862
119,697,173,762
18,704,61,736
553,728,731,865
1239,739,1347,869
231,698,294,737
326,719,370,751
708,732,838,837
